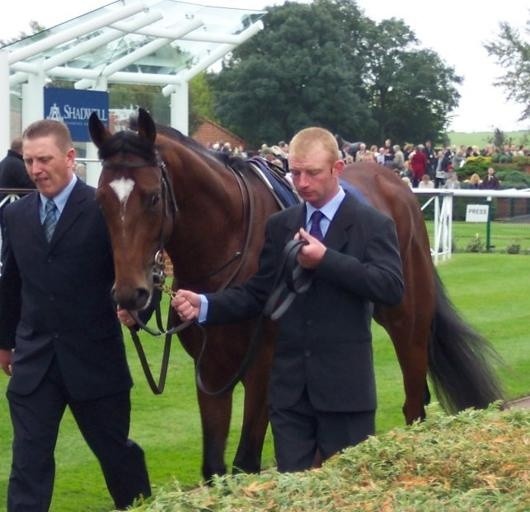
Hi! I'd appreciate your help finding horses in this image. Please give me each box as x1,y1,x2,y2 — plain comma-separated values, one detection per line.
88,107,509,487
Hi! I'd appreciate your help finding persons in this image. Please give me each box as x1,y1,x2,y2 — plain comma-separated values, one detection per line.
0,119,164,512
169,126,404,474
0,137,37,241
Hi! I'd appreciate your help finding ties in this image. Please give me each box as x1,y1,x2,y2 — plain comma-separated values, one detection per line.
41,199,57,244
308,211,327,245
